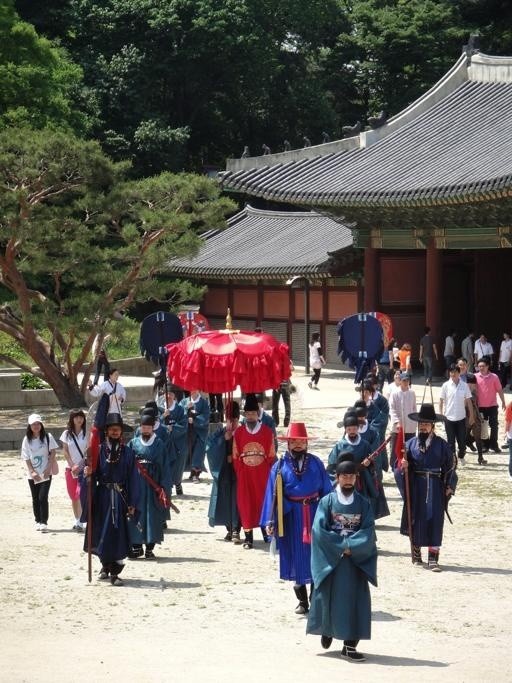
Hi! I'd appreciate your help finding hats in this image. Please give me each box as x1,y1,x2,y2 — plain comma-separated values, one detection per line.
275,422,320,440
244,393,259,412
225,401,240,420
104,400,159,432
408,403,447,422
337,372,378,428
28,413,43,424
400,371,408,380
326,451,363,474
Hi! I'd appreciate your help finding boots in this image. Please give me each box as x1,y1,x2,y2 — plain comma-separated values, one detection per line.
411,545,423,565
428,547,441,571
320,634,332,647
98,561,125,586
293,584,309,613
341,638,364,661
128,543,143,559
174,483,182,495
191,467,201,483
146,543,157,560
224,528,252,549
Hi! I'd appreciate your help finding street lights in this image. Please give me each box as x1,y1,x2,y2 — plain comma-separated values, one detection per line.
286,274,311,374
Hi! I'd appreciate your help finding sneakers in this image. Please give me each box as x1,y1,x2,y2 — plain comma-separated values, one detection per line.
72,522,83,532
459,441,509,465
36,522,47,532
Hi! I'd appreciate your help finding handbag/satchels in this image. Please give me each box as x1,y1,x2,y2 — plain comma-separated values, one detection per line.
471,419,491,441
48,458,59,476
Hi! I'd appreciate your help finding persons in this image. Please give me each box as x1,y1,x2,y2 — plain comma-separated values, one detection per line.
21,414,59,531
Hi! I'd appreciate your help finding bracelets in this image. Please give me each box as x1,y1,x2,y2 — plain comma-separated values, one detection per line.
31,471,34,473
